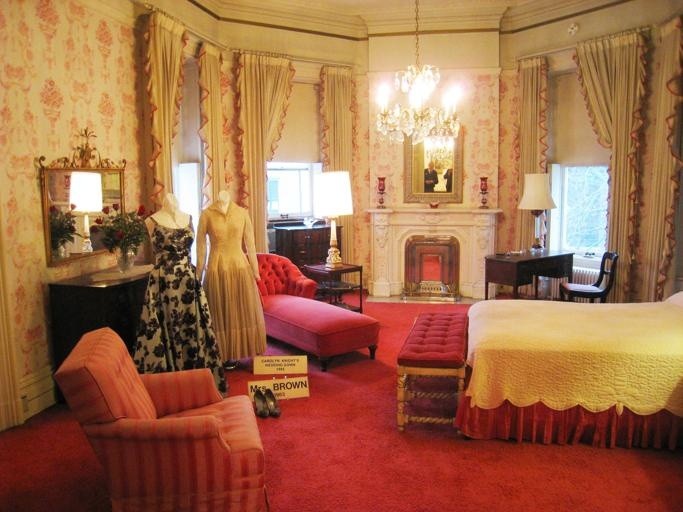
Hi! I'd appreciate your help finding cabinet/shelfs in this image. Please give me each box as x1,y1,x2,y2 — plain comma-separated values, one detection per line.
274,229,343,303
306,262,362,315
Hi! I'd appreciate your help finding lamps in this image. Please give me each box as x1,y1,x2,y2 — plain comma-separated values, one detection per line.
372,0,465,145
312,171,353,270
68,170,103,251
516,173,556,254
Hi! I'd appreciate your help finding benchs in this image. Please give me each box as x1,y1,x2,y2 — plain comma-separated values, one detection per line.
396,312,468,432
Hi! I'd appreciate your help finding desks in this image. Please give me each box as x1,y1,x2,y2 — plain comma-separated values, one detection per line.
47,263,155,407
484,251,574,300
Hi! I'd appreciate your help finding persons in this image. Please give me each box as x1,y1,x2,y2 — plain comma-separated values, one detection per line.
134,193,229,397
443,168,452,192
196,191,266,369
424,161,439,193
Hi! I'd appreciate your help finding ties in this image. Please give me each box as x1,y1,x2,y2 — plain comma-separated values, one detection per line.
429,170,431,174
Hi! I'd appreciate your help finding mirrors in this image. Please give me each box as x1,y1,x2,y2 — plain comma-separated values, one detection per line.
40,168,126,268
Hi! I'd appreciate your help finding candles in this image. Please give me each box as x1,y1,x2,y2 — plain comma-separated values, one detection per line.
84,214,89,232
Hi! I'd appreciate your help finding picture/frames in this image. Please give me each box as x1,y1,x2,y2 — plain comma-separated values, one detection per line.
403,125,464,205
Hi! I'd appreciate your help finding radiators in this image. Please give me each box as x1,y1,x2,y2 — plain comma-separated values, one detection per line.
550,265,606,302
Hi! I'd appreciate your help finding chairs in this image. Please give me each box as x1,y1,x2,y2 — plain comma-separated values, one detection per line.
245,253,379,372
559,250,618,302
50,325,268,512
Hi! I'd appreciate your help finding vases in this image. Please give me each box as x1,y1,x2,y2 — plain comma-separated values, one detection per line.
53,245,69,259
116,252,135,274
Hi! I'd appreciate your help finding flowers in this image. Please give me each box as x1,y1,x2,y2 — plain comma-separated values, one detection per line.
48,203,77,257
97,204,148,274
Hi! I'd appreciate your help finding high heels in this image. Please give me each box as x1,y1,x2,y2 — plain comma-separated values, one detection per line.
264,389,280,417
253,389,269,418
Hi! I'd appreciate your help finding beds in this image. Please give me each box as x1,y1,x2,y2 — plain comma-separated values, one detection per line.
452,290,683,453
